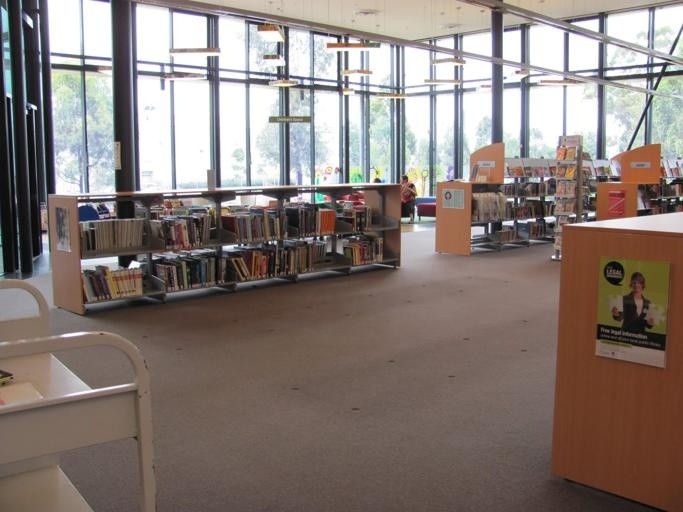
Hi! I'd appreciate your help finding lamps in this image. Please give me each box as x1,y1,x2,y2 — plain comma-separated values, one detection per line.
257,0,285,43
263,1,285,66
327,0,380,51
169,48,220,57
342,7,372,75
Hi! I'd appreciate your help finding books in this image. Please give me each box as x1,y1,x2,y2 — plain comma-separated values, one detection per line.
580,151,621,225
502,157,556,243
636,156,683,216
469,191,505,244
553,146,576,258
77,195,383,301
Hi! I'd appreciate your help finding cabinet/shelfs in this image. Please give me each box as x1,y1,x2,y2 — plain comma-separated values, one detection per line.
552,212,683,512
596,182,683,221
618,143,683,182
47,183,403,315
436,182,597,256
470,143,622,184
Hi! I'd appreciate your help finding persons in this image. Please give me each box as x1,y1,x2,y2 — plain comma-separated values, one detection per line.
58,214,69,253
400,175,417,224
374,178,382,183
612,273,654,333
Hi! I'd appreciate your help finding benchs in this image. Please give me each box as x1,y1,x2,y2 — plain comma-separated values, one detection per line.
415,197,436,207
417,202,437,221
